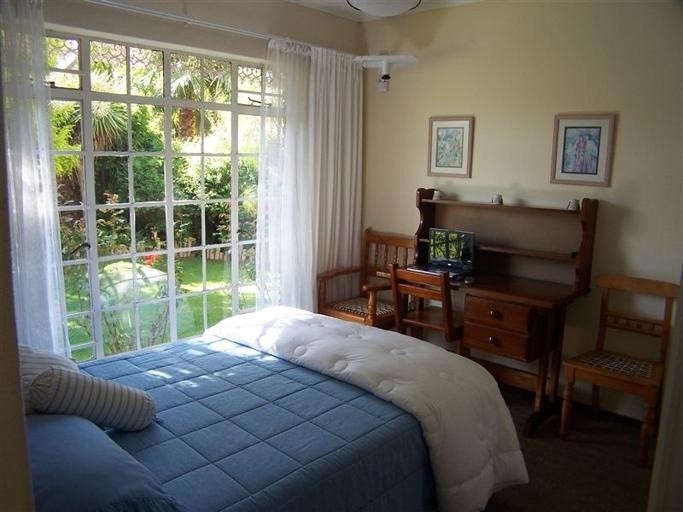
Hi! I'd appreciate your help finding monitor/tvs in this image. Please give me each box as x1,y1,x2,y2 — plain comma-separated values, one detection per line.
427,227,474,272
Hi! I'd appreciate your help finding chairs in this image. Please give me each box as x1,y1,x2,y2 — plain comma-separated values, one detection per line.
316,225,416,330
559,271,682,467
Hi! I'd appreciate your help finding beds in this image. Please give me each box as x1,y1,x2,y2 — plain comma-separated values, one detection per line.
22,332,485,511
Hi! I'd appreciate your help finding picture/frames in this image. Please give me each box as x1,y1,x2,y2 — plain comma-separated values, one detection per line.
426,114,474,180
549,111,619,188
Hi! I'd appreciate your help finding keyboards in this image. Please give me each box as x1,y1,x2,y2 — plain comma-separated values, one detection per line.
406,265,461,279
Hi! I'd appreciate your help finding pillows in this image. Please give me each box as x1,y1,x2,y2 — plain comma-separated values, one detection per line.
15,341,186,511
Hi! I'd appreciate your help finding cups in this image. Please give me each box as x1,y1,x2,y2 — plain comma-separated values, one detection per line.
566,198,580,211
491,193,504,205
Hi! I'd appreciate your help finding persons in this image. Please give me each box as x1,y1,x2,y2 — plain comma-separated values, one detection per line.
584,134,598,173
574,134,583,175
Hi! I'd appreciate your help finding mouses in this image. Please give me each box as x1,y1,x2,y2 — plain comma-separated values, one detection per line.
464,278,475,285
